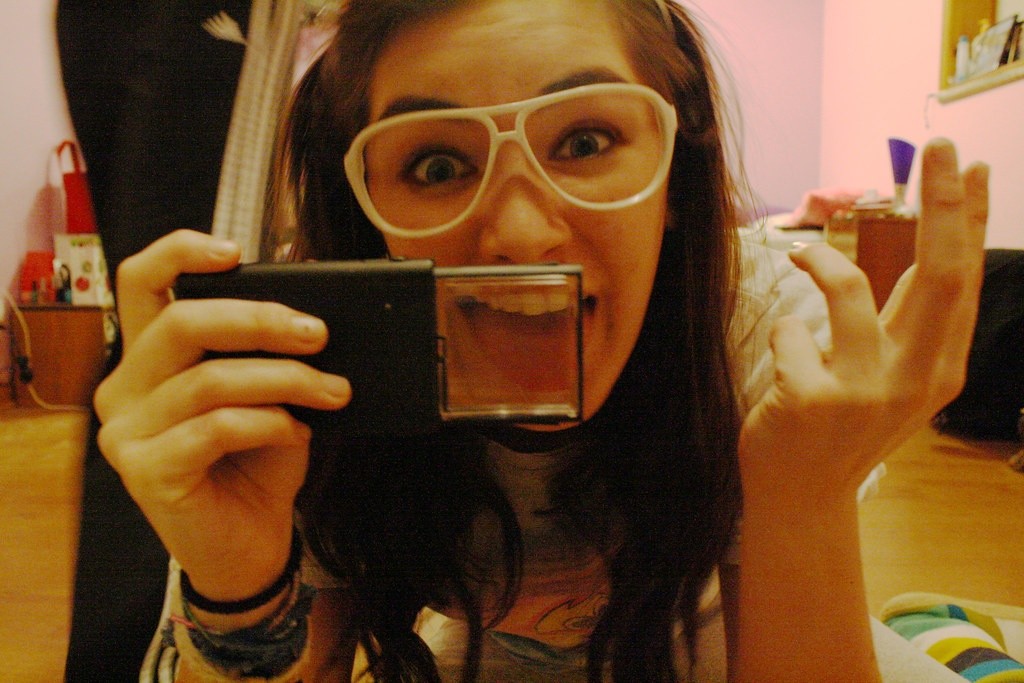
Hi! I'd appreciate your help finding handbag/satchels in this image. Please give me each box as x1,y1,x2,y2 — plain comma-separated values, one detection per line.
57,141,96,235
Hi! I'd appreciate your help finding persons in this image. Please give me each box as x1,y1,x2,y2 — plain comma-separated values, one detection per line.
57,0,255,682
94,0,988,683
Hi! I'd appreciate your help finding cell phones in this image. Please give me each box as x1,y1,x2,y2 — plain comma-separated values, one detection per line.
175,255,587,442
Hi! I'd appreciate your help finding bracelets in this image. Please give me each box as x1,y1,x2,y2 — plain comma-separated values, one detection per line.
169,527,316,683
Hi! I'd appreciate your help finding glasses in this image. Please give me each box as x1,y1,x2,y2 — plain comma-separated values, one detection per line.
344,82,678,238
